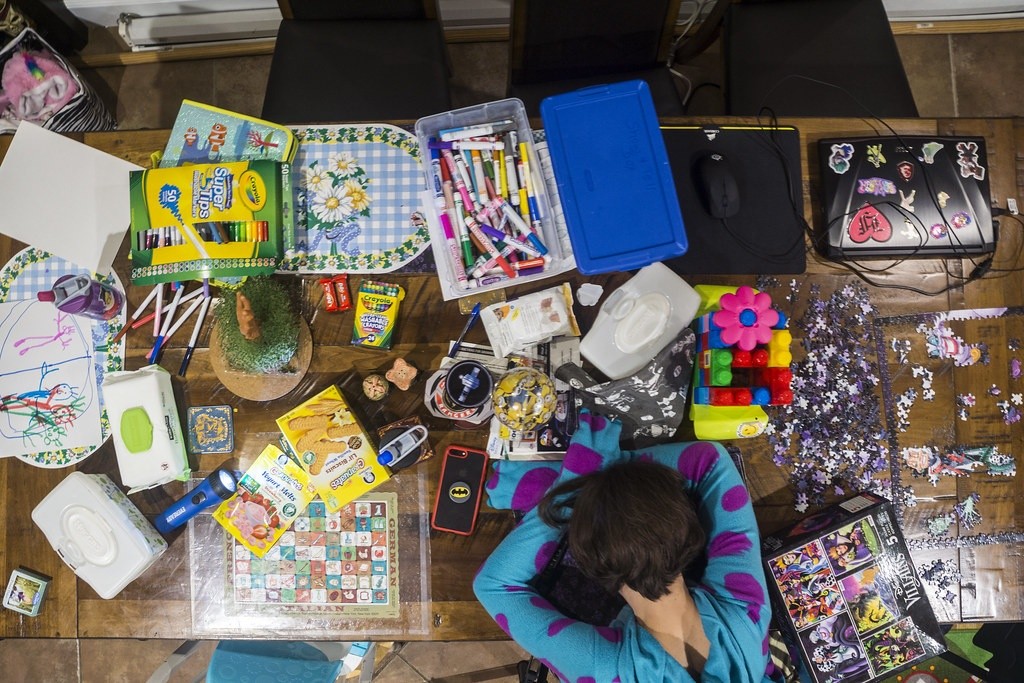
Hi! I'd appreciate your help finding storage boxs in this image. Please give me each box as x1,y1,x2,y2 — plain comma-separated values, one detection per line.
0,26,120,132
757,491,948,683
413,97,566,296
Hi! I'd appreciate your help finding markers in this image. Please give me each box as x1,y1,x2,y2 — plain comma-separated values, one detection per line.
425,118,552,293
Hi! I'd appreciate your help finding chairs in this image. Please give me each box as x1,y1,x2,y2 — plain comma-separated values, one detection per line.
505,0,687,118
260,0,453,124
671,0,920,118
145,637,376,683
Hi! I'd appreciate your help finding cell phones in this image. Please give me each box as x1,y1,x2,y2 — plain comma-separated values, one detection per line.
431,445,488,536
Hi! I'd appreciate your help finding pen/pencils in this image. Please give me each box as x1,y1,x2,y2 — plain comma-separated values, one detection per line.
113,278,212,377
447,302,481,358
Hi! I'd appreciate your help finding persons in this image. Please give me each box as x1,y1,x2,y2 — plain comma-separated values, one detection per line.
473,406,777,683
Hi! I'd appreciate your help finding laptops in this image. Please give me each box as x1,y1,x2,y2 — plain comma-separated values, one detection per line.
816,135,995,262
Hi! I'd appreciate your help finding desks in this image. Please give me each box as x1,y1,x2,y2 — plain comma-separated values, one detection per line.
1,117,1024,640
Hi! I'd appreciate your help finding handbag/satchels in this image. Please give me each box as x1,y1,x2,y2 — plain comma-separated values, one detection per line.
0,24,118,132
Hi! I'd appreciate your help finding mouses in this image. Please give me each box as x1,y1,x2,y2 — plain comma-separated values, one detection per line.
691,153,739,219
